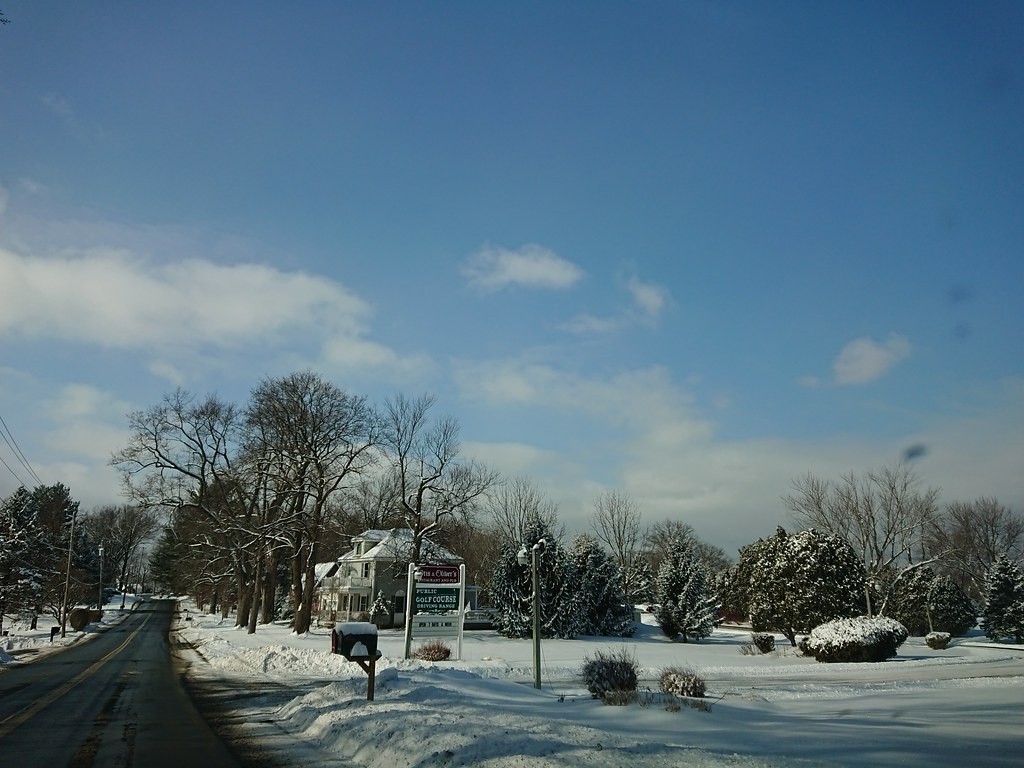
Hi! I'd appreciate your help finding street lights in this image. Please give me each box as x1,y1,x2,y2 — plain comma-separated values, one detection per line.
403,567,423,659
518,544,542,688
